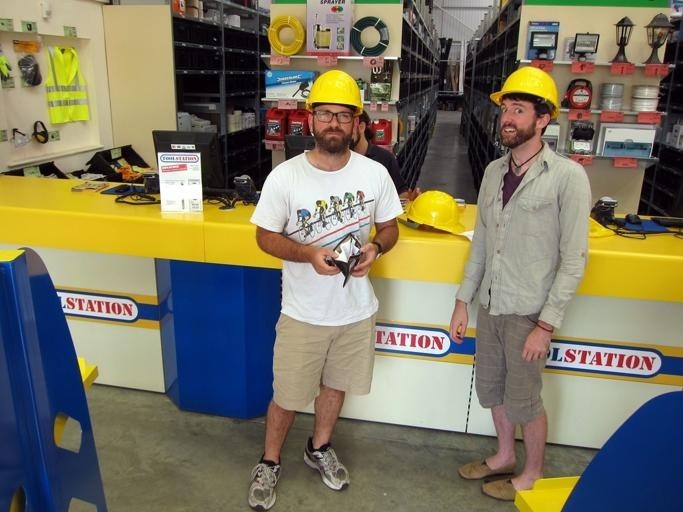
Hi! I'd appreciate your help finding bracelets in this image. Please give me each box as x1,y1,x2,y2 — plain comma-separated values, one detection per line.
536,323,554,336
370,241,381,254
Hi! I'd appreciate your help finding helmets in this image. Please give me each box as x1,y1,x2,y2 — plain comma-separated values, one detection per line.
305,70,363,115
407,188,465,235
489,67,560,119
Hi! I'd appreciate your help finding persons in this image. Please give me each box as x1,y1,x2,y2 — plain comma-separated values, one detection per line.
244,65,406,510
348,107,423,203
447,65,594,505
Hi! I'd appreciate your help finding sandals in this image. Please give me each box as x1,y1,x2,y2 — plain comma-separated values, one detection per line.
458,459,515,479
481,476,530,501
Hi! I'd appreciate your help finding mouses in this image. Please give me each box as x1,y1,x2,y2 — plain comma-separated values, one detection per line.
626,214,641,224
115,185,130,193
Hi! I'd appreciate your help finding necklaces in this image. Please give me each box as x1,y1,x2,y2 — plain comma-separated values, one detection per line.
509,144,542,174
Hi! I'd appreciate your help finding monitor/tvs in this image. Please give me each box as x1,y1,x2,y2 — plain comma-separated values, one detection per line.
152,130,228,201
283,135,354,160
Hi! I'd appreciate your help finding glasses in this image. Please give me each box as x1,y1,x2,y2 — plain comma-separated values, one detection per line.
312,109,355,123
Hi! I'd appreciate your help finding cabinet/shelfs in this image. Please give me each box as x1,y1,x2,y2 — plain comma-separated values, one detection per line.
260,1,445,199
462,3,673,214
0,28,105,167
102,3,270,201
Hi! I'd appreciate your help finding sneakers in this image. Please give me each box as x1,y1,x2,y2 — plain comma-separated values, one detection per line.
303,438,351,492
247,455,281,510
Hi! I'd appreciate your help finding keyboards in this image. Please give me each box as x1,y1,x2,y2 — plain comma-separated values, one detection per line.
651,218,683,227
202,187,236,197
